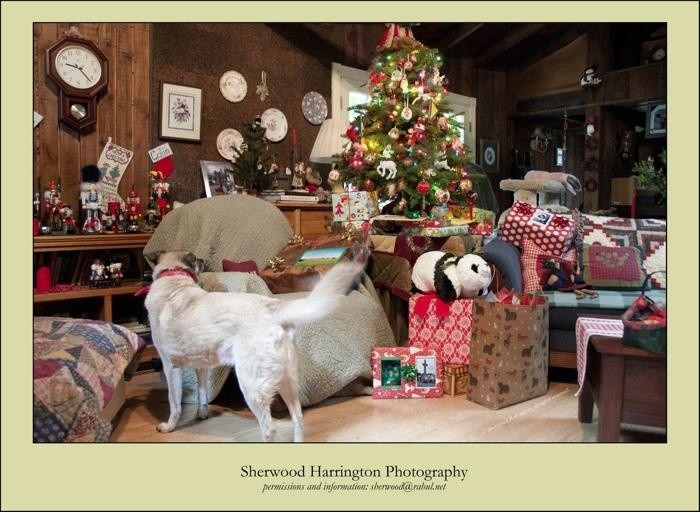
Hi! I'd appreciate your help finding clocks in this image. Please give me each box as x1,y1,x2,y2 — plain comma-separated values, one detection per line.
46,37,109,131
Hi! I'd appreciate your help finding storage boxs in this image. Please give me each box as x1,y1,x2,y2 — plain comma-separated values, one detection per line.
497,200,576,258
408,292,473,365
611,177,656,205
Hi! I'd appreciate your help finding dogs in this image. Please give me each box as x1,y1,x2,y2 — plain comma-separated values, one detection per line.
141,245,373,442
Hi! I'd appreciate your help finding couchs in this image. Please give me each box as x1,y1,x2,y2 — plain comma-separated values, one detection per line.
480,213,666,369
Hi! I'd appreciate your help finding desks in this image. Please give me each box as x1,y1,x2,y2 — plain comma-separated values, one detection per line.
610,205,666,216
368,233,484,346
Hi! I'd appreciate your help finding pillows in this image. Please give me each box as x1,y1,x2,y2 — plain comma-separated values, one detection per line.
520,235,576,295
578,244,648,290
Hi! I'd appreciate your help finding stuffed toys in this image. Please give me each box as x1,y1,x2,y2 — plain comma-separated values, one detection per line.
410,251,493,302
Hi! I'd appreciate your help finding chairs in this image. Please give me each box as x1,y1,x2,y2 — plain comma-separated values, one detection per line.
143,194,398,419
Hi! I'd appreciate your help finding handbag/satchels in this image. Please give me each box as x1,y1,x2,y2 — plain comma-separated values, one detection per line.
621,295,667,355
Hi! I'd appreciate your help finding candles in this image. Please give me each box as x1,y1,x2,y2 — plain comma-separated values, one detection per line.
292,128,296,145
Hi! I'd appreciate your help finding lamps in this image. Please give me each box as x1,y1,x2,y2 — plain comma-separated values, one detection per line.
309,119,351,164
530,123,552,154
579,67,602,101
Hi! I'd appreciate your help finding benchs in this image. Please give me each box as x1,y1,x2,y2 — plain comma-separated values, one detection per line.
34,316,148,442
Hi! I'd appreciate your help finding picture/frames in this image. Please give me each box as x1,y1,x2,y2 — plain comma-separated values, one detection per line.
200,160,237,197
370,346,444,399
645,101,667,139
480,138,500,174
159,79,205,143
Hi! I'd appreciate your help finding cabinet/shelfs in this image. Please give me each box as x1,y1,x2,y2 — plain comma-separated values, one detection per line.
33,233,160,370
271,200,333,238
